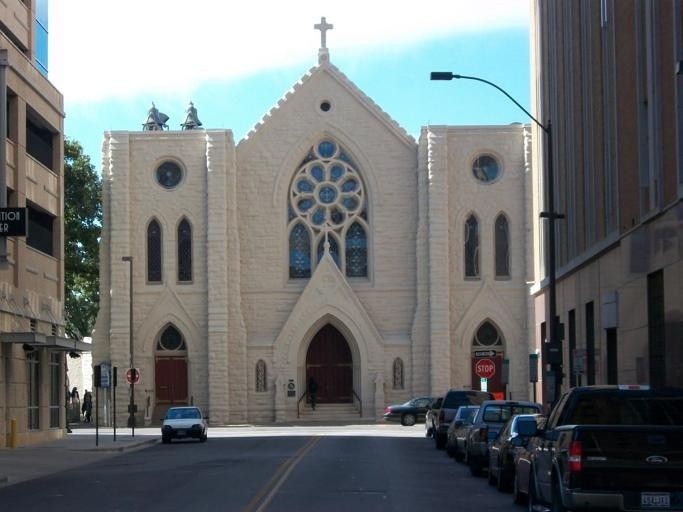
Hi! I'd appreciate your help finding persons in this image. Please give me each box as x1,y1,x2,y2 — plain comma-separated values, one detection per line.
309,375,317,410
146,101,158,123
69,387,92,422
184,100,197,124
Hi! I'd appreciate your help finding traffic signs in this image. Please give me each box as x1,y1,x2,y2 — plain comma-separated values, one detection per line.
474,350,496,358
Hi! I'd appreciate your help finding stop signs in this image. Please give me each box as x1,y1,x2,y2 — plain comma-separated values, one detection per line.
474,358,495,378
125,367,140,384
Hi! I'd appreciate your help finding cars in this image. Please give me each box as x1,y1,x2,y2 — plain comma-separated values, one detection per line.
382,384,681,509
161,407,207,443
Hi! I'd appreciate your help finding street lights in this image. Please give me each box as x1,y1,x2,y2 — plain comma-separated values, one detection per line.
121,256,134,436
431,70,564,395
